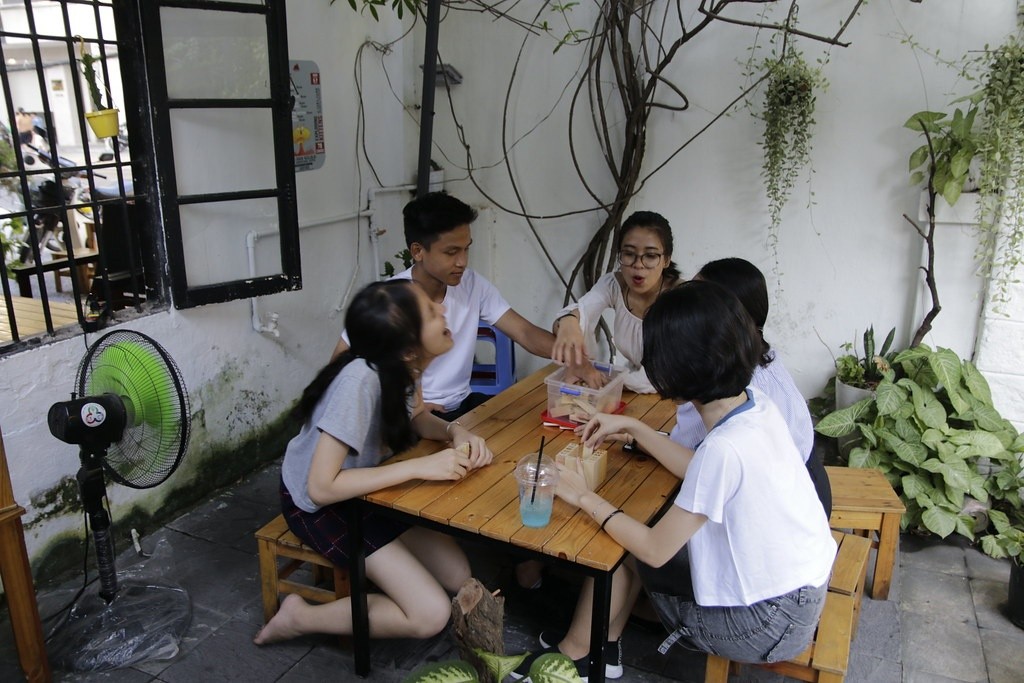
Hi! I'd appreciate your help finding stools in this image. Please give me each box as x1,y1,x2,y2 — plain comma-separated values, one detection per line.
829,530,871,639
825,465,905,603
255,512,335,615
704,591,854,683
467,320,519,400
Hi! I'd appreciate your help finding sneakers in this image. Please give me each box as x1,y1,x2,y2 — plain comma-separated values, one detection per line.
510,642,590,683
539,630,623,679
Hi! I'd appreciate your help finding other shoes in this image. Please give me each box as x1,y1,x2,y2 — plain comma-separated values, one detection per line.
508,571,577,625
628,592,667,635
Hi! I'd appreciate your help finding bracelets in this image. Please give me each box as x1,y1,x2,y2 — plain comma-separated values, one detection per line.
601,510,624,533
593,501,608,520
446,421,461,440
557,314,577,327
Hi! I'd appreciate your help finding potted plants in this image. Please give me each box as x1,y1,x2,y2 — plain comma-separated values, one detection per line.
86,56,119,140
429,161,445,191
835,322,897,408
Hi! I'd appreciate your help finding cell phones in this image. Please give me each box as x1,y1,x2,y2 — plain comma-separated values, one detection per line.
622,431,670,452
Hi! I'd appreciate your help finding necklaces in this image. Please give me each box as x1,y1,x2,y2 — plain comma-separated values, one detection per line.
626,275,665,312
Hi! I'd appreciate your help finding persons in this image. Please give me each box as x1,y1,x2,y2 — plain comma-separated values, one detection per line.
552,211,686,394
510,258,839,683
254,192,609,646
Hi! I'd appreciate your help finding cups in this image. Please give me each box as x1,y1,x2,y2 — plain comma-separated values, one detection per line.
514,454,558,529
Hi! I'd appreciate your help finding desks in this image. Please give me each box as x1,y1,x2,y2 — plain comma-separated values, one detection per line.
343,363,678,683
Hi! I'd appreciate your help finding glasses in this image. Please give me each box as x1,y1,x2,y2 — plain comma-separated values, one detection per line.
618,247,665,269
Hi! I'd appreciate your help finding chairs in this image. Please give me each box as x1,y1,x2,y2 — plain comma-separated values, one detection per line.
50,220,97,292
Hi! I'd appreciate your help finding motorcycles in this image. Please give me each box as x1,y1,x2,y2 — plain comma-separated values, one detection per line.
14,126,136,283
106,121,129,152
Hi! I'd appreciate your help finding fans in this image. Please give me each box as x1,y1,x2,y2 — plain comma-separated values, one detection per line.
43,328,190,668
